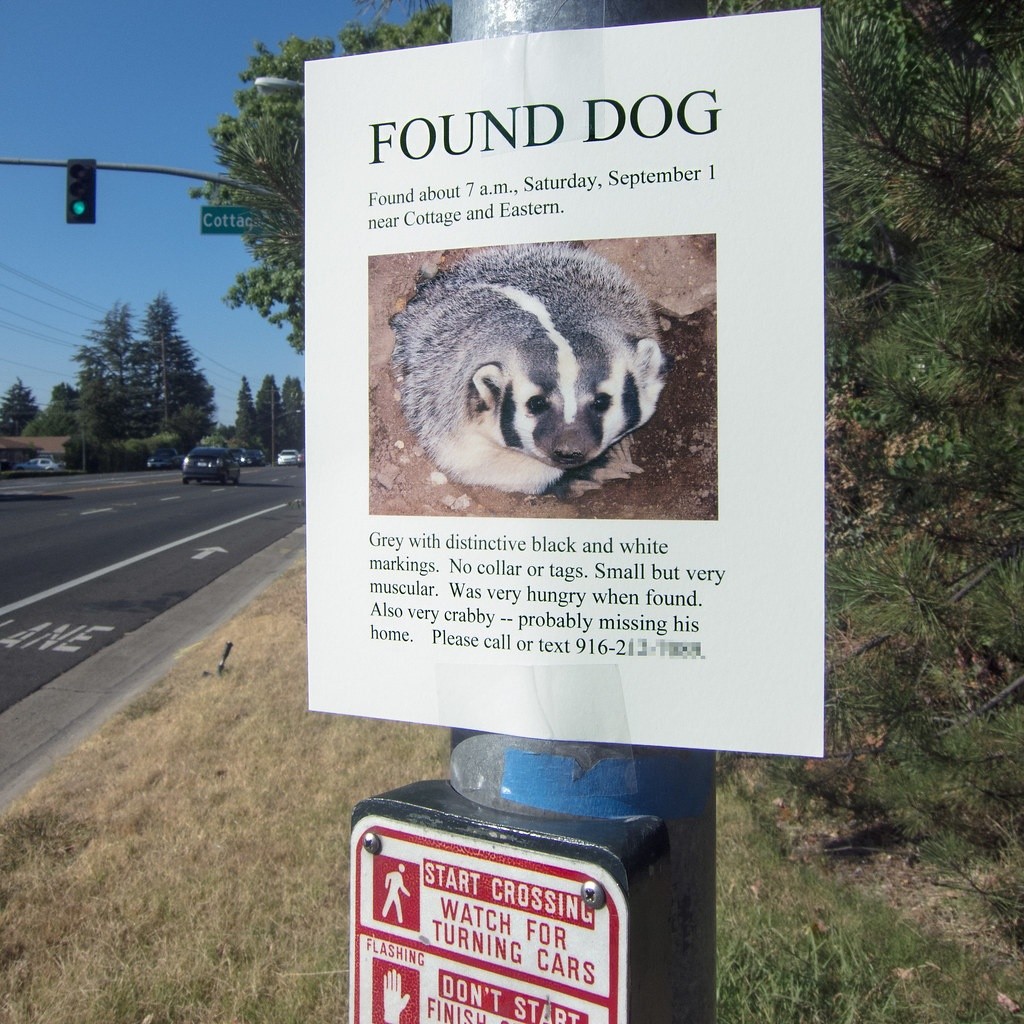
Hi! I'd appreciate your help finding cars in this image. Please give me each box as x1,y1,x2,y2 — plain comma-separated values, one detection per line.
13,458,65,471
228,448,266,467
146,448,186,469
277,449,305,467
182,447,241,485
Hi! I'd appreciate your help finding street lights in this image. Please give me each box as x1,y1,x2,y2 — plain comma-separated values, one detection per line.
254,77,304,92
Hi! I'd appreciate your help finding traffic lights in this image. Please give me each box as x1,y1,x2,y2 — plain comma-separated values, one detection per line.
66,158,96,224
